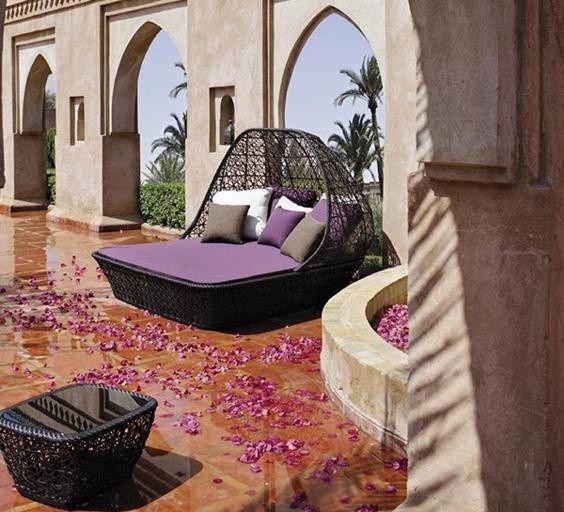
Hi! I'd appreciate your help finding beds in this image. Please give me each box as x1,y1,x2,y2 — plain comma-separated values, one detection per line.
89,128,375,333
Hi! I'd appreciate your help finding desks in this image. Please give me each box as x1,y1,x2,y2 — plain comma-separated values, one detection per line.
1,380,160,512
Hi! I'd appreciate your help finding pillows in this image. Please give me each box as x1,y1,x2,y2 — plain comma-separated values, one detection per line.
198,185,363,264
